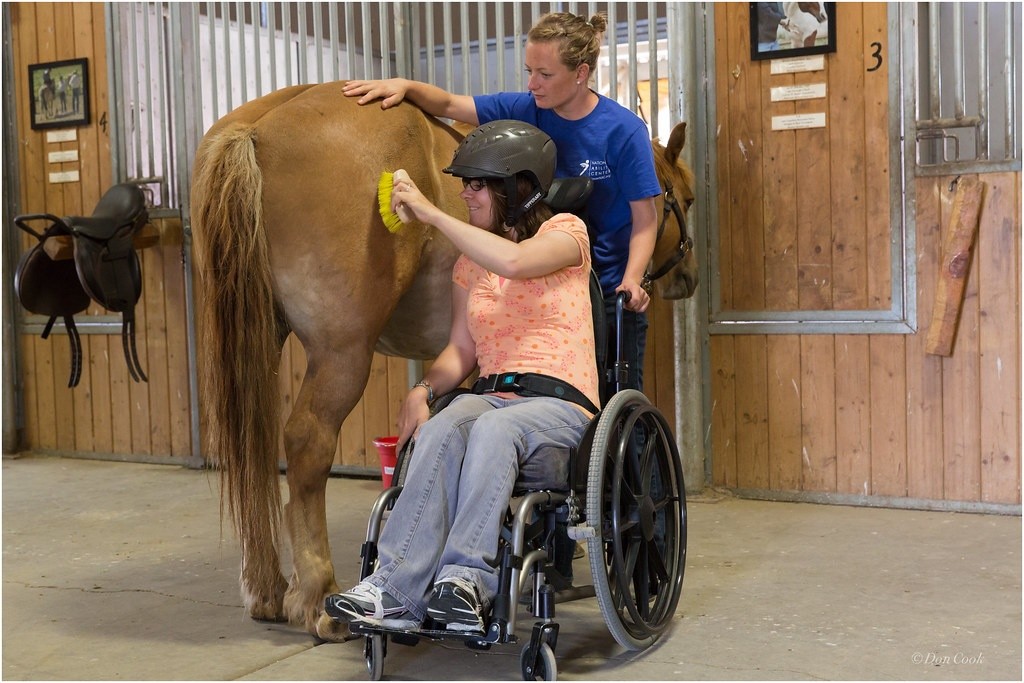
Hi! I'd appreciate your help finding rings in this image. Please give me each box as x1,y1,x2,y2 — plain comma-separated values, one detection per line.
406,185,410,192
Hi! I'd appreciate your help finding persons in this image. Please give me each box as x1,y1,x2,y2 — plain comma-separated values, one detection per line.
757,2,798,52
326,120,602,635
69,70,81,113
43,68,52,85
56,76,66,112
342,12,663,605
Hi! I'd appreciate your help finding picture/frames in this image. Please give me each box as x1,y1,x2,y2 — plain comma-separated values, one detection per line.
26,56,95,133
748,2,840,62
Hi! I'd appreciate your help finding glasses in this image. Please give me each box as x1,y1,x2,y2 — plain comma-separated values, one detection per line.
461,177,488,191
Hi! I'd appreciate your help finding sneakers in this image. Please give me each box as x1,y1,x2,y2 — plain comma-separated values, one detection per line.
324,579,421,631
425,577,485,632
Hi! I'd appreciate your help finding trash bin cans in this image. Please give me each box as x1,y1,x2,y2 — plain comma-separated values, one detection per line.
373,435,401,489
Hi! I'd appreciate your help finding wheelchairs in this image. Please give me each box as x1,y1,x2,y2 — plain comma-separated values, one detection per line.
349,290,690,682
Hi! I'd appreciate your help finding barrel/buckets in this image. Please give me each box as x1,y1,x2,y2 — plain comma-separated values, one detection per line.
372,436,398,490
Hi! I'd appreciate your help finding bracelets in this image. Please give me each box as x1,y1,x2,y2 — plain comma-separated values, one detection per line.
413,381,433,400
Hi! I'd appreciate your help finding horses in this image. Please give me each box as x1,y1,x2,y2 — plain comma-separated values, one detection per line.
187,80,700,644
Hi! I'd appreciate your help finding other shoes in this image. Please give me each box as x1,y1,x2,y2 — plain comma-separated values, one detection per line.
520,568,574,604
610,574,656,605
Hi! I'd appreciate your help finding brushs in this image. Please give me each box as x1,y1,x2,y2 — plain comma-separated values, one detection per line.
376,169,415,233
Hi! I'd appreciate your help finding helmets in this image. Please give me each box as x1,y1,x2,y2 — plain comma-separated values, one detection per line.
442,120,558,192
